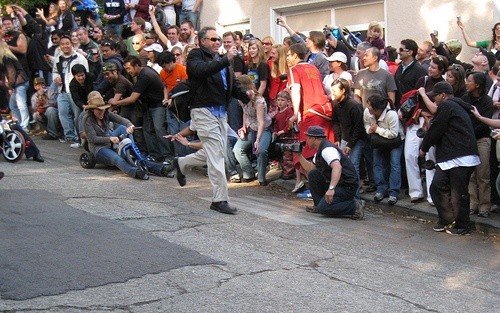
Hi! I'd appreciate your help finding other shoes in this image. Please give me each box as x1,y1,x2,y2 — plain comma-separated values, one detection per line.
445,226,473,236
432,219,455,231
23,125,490,221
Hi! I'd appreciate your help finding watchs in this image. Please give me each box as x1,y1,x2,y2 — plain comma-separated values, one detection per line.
329,186,334,189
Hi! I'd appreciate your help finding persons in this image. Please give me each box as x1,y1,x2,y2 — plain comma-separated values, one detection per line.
0,0,500,217
296,126,364,221
420,82,481,235
173,26,238,214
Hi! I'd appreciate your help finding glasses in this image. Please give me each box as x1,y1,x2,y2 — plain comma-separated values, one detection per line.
131,41,141,45
204,37,221,42
336,77,345,88
92,30,99,33
399,48,409,52
479,52,486,59
261,42,272,46
100,42,114,46
452,63,462,77
435,54,445,66
173,53,181,57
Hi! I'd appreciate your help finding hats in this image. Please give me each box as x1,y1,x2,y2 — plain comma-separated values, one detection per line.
479,47,496,71
82,90,111,110
102,63,117,71
32,77,46,86
445,39,462,58
143,43,163,53
425,81,453,96
303,125,327,138
325,51,347,63
243,34,253,40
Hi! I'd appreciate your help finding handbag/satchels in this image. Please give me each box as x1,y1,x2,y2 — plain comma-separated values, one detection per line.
370,109,401,149
400,76,429,119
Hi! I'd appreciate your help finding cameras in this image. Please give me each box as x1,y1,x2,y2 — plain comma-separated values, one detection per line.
433,30,438,36
457,16,461,21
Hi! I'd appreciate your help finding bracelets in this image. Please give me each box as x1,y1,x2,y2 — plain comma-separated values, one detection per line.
188,142,190,146
